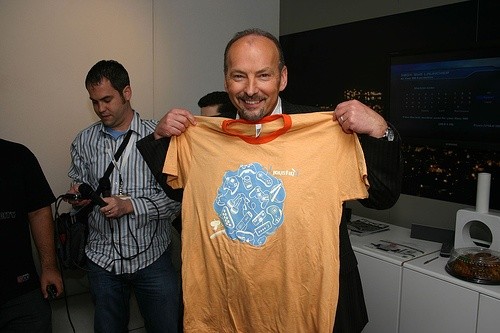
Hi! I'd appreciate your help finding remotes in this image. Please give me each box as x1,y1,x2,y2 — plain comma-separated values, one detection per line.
440,239,454,257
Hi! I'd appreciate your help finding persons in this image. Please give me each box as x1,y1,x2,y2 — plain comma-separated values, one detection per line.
198,91,237,120
62,60,182,333
0,138,63,333
134,28,402,333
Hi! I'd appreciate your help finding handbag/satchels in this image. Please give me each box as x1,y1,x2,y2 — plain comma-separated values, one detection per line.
53,212,89,279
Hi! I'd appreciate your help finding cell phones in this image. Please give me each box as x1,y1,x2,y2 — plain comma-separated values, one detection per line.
47,285,57,298
66,193,82,200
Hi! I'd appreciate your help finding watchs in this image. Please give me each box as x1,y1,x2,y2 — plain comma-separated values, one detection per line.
371,127,395,142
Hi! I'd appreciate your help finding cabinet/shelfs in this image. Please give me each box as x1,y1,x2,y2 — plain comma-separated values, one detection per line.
398,251,500,333
348,215,442,333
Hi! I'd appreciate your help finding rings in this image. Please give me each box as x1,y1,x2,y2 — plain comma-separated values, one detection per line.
340,115,345,122
108,210,111,214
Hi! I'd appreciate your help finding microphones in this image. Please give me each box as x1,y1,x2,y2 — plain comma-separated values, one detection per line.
100,177,113,232
78,183,108,208
